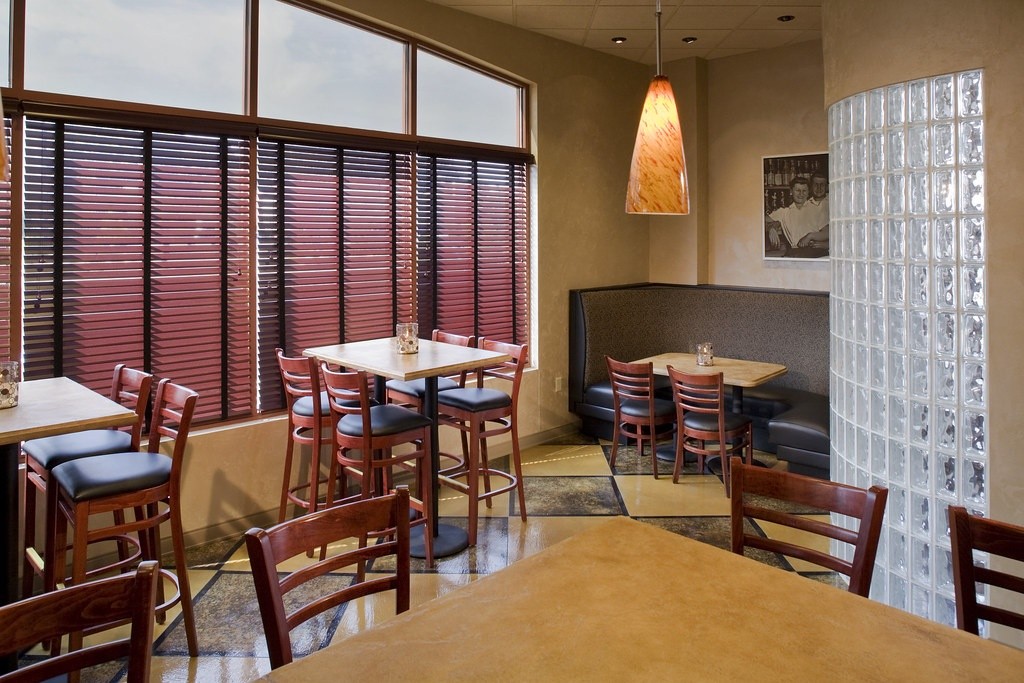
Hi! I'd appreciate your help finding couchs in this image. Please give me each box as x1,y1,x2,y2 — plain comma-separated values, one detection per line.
569,281,829,482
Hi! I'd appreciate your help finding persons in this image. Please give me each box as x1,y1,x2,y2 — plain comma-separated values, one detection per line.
765,170,829,258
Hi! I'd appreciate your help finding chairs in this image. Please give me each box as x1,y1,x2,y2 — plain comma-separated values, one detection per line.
244,484,410,671
948,505,1024,636
667,365,754,497
603,354,677,479
717,456,889,599
276,328,528,564
0,365,200,683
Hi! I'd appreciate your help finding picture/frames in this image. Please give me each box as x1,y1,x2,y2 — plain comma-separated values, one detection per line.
761,151,829,262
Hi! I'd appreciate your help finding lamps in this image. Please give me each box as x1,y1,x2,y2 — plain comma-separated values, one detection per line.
624,0,690,215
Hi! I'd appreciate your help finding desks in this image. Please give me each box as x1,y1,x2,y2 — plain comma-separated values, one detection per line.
302,336,514,558
256,515,1024,683
628,352,788,476
0,376,140,676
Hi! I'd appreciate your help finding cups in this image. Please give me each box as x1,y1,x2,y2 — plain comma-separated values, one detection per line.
696,342,714,366
0,360,19,409
395,322,419,355
